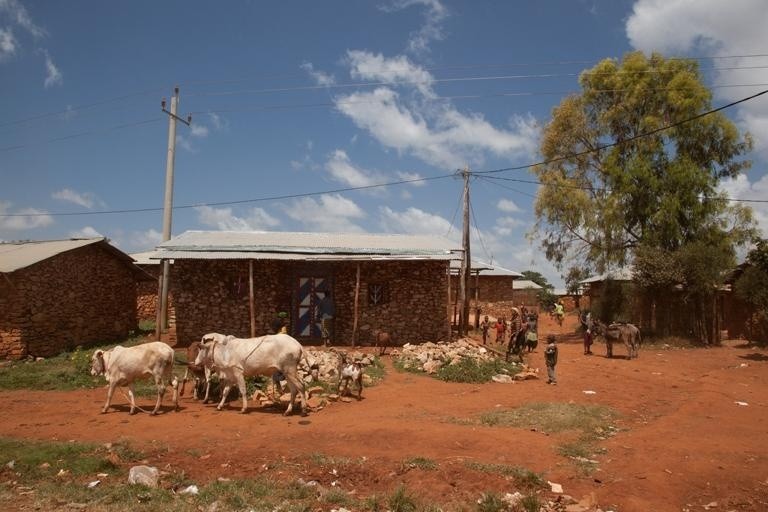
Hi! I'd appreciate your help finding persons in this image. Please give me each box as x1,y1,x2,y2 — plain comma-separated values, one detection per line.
544,333,558,385
554,301,565,327
319,292,335,348
583,327,594,355
263,309,288,396
477,299,540,357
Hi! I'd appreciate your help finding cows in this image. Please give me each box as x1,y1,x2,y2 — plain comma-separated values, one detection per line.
90,341,180,416
179,331,319,417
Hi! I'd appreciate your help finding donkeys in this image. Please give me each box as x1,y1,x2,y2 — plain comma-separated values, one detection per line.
579,311,642,361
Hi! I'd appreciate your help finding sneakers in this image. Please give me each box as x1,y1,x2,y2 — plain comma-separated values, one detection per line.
582,348,594,357
544,378,558,386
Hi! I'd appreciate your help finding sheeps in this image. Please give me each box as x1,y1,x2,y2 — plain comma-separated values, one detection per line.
335,351,363,402
373,328,394,357
